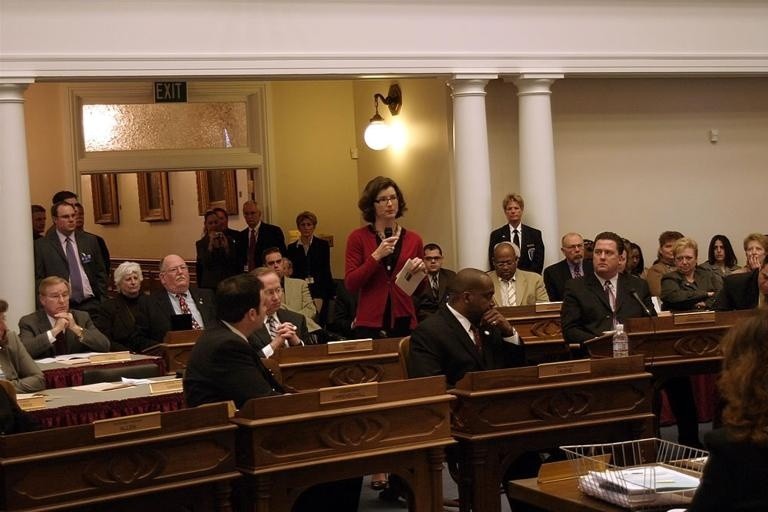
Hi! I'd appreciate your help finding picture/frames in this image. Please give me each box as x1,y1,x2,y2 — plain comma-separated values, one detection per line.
137,172,171,222
196,170,239,217
91,174,120,225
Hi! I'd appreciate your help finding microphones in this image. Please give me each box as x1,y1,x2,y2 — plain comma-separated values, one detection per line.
629,288,651,316
385,227,392,271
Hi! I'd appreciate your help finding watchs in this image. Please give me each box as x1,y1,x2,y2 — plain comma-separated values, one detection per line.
501,326,516,338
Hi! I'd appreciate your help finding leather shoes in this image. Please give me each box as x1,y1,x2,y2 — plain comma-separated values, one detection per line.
371,473,388,490
678,436,705,450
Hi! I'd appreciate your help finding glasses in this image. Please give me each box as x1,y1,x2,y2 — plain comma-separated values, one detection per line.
493,259,515,268
375,197,399,206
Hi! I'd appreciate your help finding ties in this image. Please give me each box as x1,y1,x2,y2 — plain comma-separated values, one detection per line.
65,236,84,304
430,272,439,301
267,317,280,340
574,266,581,278
55,331,65,354
175,293,201,330
507,279,517,307
513,230,519,248
470,325,483,357
248,228,256,272
605,282,619,324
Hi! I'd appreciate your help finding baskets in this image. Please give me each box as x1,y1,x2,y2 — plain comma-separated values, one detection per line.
560,437,710,508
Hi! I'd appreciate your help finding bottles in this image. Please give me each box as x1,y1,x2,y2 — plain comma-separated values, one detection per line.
613,324,628,357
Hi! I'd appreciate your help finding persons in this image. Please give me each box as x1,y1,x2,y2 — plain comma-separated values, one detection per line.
182,274,364,512
344,177,429,340
411,231,768,325
489,194,545,274
1,191,333,395
409,268,545,512
683,312,768,512
561,231,708,459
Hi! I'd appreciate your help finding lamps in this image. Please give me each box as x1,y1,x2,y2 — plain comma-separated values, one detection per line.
364,86,403,151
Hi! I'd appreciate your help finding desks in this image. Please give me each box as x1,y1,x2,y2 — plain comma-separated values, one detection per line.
0,299,767,511
